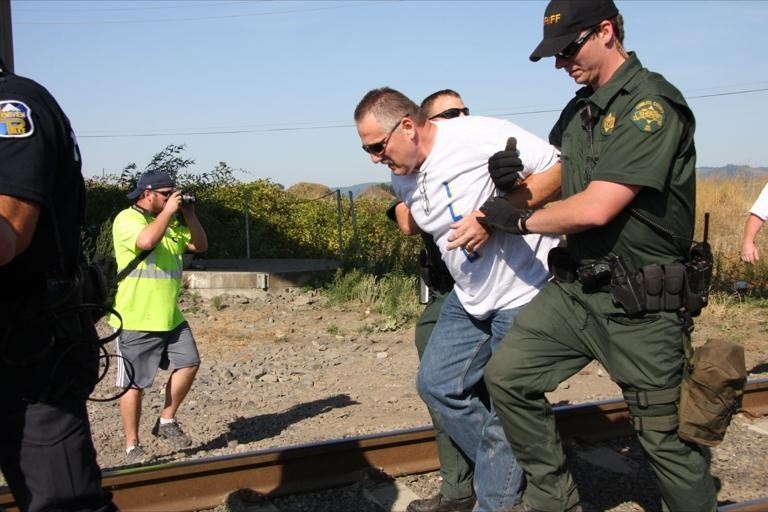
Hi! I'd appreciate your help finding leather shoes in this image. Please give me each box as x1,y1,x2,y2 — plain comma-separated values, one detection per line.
406,493,476,512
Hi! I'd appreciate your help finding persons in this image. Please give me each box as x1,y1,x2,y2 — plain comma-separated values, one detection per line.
0,72,105,510
386,90,477,512
354,87,562,512
107,171,209,458
740,176,766,268
477,3,717,510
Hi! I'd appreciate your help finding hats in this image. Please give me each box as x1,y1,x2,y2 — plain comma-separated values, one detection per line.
530,0,618,62
126,170,174,199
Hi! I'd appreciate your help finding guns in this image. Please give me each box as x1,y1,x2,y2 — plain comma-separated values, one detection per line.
577,251,642,314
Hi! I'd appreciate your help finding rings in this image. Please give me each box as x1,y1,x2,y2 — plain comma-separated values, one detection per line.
467,242,473,251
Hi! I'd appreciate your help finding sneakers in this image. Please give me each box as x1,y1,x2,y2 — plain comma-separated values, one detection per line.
124,438,156,464
151,417,192,448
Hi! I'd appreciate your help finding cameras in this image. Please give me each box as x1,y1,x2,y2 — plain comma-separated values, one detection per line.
178,190,198,208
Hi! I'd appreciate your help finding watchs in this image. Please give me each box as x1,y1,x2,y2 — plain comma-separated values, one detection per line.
520,205,534,237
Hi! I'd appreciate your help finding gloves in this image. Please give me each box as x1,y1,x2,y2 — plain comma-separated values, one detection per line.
488,137,524,192
477,197,526,235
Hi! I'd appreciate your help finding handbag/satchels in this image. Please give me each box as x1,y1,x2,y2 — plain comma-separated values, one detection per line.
676,339,747,449
92,253,117,310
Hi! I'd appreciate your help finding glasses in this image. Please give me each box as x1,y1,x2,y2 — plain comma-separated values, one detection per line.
555,27,597,59
428,108,469,119
152,191,172,197
362,114,410,153
